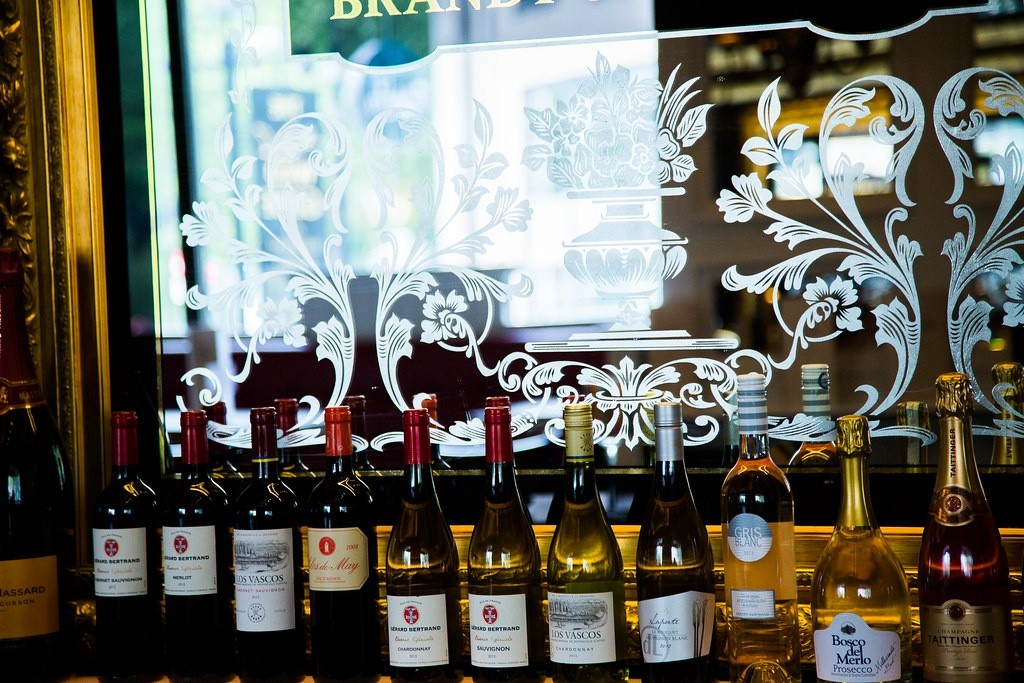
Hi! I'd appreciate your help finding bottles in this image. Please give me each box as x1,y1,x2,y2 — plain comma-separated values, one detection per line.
0,246,74,683
161,410,233,682
233,407,306,682
788,365,842,473
722,374,801,683
544,402,630,683
986,360,1021,472
385,409,463,683
204,402,242,478
413,394,452,473
544,394,589,525
347,394,380,475
636,401,720,683
627,392,657,528
304,406,382,683
87,410,163,683
812,416,913,683
894,402,926,473
919,372,1012,683
274,400,315,476
468,408,546,683
486,397,532,522
723,385,739,470
